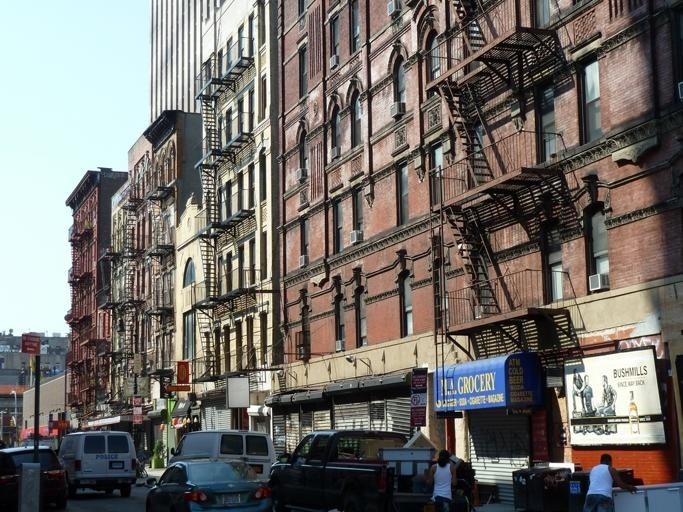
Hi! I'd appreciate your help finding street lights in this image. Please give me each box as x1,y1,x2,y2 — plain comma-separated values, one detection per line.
8,390,17,447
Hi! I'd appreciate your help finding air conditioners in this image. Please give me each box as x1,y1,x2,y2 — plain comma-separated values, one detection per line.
386,0,401,17
588,271,609,293
295,168,306,181
328,54,338,70
298,254,308,268
389,101,405,119
349,229,362,245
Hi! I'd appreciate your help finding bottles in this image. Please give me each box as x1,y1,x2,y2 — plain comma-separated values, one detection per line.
628,391,641,436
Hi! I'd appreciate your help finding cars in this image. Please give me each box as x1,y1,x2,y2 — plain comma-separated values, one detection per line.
141,459,268,511
2,446,68,510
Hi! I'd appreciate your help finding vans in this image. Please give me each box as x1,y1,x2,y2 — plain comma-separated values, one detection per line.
169,431,277,488
59,429,139,499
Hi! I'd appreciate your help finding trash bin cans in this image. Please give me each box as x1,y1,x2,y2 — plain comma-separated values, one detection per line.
512,466,633,512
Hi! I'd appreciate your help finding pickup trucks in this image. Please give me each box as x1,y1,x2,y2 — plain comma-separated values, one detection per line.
266,430,465,511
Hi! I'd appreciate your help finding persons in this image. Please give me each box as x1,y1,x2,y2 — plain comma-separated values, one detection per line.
583,452,637,511
594,375,617,417
582,374,597,415
570,369,585,416
425,450,456,512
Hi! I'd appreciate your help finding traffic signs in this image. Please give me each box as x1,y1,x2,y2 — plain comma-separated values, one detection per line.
21,334,40,354
